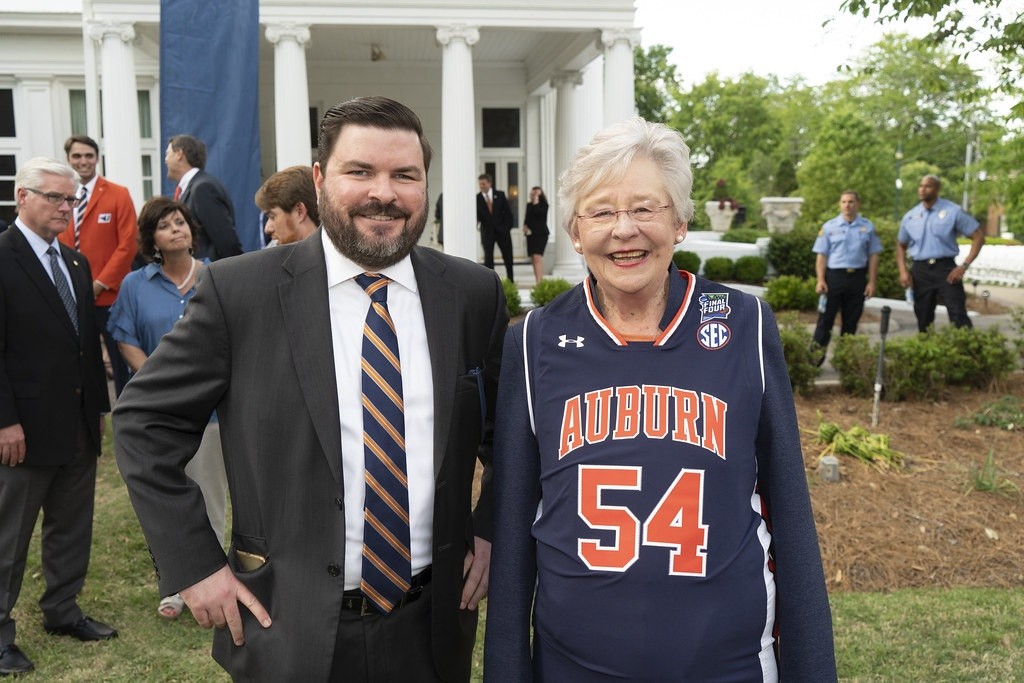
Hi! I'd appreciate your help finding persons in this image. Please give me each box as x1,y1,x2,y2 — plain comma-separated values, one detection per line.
810,191,883,367
112,96,511,683
483,117,837,683
254,166,321,245
435,174,548,282
57,134,138,416
0,158,112,672
107,196,228,616
165,135,245,262
896,173,985,333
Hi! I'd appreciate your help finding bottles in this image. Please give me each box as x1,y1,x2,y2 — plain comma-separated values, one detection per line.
817,292,828,314
905,285,915,305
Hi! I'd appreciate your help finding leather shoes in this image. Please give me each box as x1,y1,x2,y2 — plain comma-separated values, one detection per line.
44,616,118,641
0,646,35,675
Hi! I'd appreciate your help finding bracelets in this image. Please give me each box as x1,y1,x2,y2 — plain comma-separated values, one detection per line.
963,261,969,267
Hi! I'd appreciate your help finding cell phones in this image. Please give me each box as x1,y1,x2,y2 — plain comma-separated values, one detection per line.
235,550,266,570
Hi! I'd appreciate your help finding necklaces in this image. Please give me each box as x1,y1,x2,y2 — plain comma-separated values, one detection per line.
177,254,195,289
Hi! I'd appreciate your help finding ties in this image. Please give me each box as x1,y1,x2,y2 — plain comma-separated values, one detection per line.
353,272,413,618
46,245,79,337
74,187,88,253
485,191,492,214
173,185,182,203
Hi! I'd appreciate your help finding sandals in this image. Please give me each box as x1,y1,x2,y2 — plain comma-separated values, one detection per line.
158,592,184,618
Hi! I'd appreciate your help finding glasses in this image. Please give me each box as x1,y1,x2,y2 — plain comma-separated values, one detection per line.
575,200,674,226
24,188,76,207
